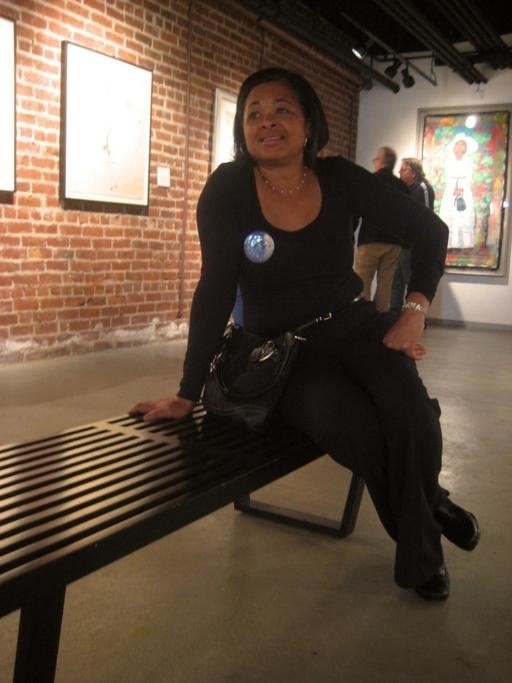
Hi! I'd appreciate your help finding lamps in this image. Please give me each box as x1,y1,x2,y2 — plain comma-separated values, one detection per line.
351,38,376,61
383,56,403,79
401,64,415,89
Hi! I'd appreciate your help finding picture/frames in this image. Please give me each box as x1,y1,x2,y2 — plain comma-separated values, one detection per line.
210,86,241,176
413,103,512,278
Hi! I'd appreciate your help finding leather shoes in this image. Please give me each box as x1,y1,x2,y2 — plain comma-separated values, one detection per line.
435,494,481,553
412,568,452,603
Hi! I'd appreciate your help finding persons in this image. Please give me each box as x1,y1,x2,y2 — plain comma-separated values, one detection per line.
440,137,477,249
130,69,480,600
352,146,434,306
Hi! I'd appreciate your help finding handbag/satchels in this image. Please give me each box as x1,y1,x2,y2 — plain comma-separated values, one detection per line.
203,326,302,437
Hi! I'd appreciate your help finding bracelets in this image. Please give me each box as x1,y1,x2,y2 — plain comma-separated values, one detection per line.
400,301,425,311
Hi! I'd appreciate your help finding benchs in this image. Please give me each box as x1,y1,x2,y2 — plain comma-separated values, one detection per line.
0,397,367,683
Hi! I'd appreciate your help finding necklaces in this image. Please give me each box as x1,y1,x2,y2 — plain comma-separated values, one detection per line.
260,166,309,194
